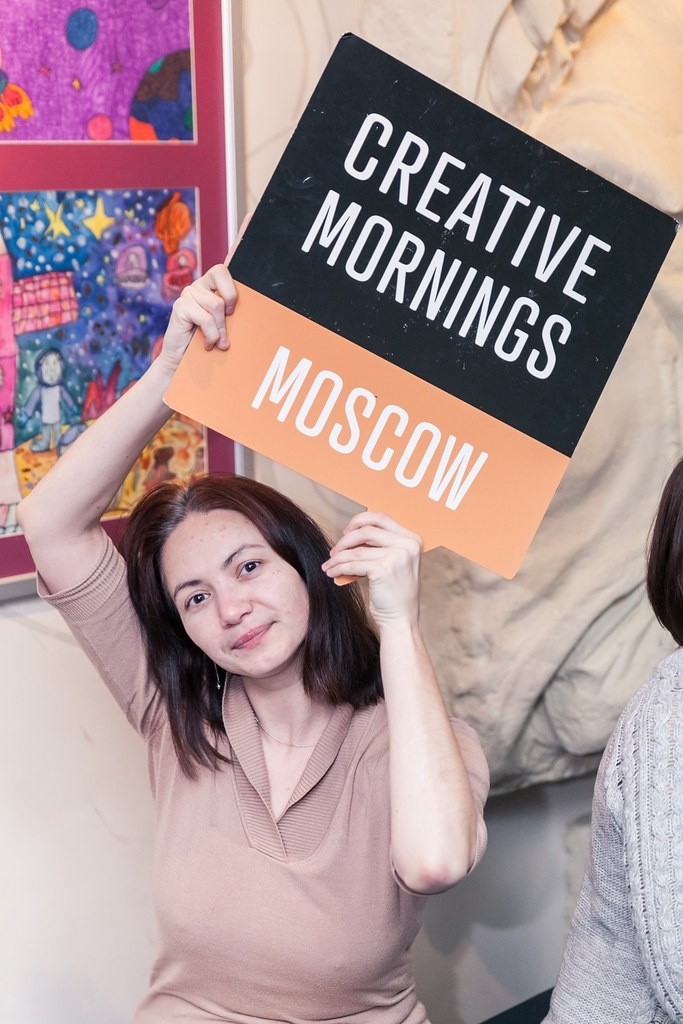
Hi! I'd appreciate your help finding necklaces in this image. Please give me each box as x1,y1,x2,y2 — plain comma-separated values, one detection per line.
255,718,318,748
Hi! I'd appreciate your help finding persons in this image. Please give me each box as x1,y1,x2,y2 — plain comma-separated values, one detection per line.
541,458,683,1024
17,265,491,1024
